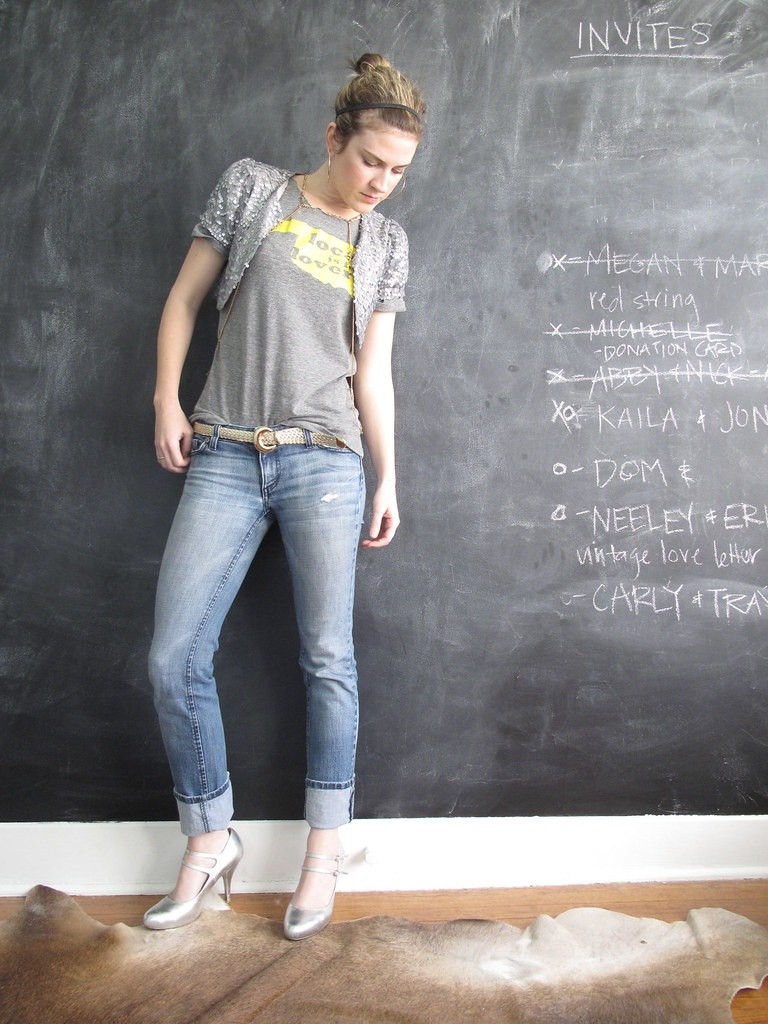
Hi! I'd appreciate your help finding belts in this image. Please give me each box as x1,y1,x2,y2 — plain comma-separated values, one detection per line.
193,421,349,454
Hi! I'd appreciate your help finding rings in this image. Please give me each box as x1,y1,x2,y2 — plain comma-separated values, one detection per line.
158,457,164,459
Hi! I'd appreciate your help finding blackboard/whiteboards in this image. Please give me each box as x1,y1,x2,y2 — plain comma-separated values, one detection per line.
0,0,768,825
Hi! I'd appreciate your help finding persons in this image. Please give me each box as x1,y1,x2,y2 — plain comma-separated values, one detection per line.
145,52,427,939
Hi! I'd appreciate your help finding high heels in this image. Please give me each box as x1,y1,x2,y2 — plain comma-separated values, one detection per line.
283,845,348,941
143,827,245,930
